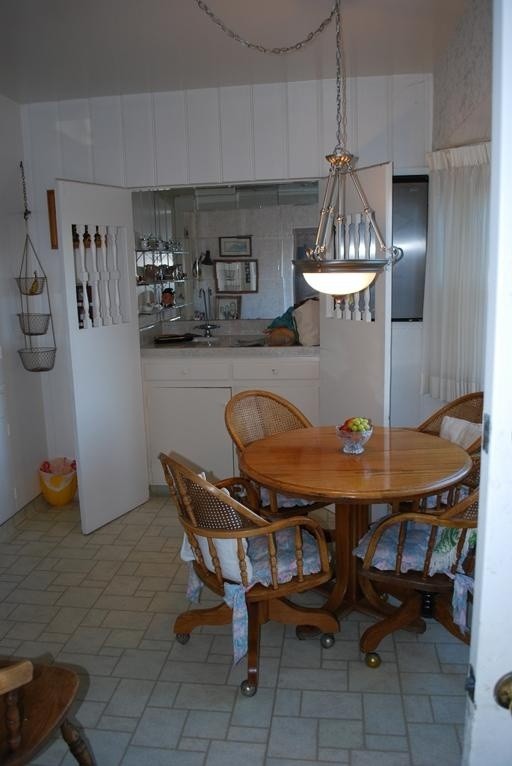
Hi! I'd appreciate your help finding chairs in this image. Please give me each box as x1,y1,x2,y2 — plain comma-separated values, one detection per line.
225,389,334,541
0,655,96,765
361,454,478,667
389,392,480,520
158,452,339,696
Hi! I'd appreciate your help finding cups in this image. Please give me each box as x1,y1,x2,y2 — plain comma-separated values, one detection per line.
139,237,185,252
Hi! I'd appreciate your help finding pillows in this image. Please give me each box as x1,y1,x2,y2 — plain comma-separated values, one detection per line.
179,470,253,584
439,415,482,451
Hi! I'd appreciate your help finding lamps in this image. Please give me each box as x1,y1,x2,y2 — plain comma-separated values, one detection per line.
291,1,398,303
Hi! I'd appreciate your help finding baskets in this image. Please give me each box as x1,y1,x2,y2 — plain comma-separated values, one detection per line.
13,278,56,371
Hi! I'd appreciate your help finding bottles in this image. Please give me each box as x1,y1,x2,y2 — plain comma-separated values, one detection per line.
143,263,174,282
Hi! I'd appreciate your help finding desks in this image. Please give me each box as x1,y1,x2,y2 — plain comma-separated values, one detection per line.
240,424,471,640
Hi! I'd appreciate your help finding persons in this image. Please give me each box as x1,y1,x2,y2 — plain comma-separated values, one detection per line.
218,302,237,321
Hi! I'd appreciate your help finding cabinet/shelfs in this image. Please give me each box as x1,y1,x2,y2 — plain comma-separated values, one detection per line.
140,347,321,497
21,75,438,190
137,249,189,315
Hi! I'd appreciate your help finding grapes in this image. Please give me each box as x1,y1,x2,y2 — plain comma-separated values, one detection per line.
348,417,370,432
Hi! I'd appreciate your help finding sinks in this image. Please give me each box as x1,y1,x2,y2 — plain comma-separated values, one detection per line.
154,335,194,343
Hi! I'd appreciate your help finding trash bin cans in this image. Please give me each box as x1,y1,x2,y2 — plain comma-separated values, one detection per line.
38,464,76,506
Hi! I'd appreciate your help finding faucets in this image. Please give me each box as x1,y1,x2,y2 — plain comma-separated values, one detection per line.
208,288,212,320
198,288,209,336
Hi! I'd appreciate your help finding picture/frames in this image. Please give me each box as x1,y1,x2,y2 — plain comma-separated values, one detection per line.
193,251,212,277
212,259,258,293
215,295,241,319
220,237,252,256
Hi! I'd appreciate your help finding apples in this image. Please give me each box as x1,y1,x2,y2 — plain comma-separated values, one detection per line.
339,425,352,438
344,418,354,426
